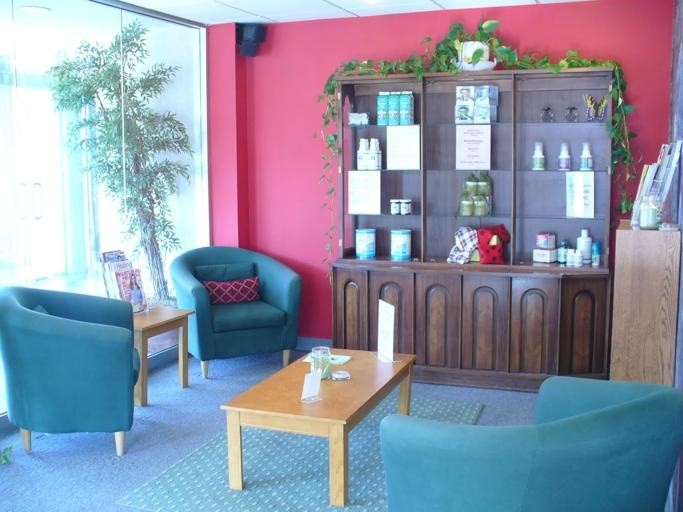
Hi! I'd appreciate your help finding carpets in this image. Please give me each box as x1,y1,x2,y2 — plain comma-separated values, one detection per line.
115,394,485,512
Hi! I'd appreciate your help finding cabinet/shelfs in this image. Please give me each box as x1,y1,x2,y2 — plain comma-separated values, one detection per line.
608,218,681,386
328,65,615,394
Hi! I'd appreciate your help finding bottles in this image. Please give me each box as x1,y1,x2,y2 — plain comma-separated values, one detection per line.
458,172,492,217
531,142,546,170
563,107,578,122
638,196,663,229
356,138,382,171
540,107,554,123
579,142,594,171
556,143,572,171
389,198,412,216
558,229,600,267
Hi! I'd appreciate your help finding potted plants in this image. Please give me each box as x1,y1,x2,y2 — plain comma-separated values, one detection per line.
313,14,644,284
42,17,194,342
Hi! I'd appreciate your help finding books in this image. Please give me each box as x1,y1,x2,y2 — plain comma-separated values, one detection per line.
631,140,682,225
101,249,149,316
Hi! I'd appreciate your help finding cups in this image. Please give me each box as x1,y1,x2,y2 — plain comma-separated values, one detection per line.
354,228,376,259
311,347,331,379
388,228,413,263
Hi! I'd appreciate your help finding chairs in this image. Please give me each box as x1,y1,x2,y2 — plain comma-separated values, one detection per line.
0,285,141,456
169,246,302,379
378,375,682,512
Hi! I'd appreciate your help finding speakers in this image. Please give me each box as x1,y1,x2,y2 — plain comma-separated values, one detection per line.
236,24,266,56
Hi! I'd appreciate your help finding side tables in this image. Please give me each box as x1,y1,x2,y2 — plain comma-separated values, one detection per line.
132,301,196,407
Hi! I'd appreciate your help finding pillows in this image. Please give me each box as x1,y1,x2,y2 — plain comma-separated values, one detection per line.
199,265,260,305
